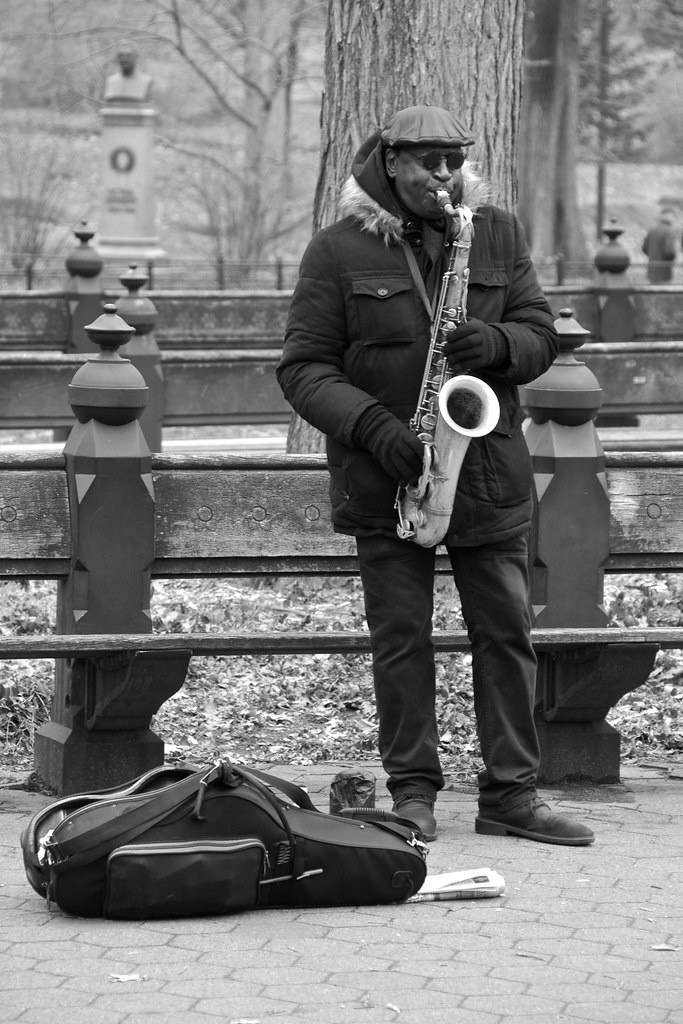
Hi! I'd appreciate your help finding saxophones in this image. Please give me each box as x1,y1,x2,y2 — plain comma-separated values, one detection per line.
392,187,501,549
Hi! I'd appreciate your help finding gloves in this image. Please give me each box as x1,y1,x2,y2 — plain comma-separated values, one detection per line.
357,406,425,487
442,316,508,374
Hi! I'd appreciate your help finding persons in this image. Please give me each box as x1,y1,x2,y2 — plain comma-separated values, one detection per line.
103,39,153,103
277,106,596,845
642,211,678,284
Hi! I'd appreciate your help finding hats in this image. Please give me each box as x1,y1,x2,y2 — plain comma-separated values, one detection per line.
379,105,475,147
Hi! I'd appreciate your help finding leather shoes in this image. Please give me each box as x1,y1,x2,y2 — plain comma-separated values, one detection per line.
475,797,595,845
392,794,437,841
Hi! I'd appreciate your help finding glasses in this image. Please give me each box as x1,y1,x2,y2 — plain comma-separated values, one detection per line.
400,145,466,170
